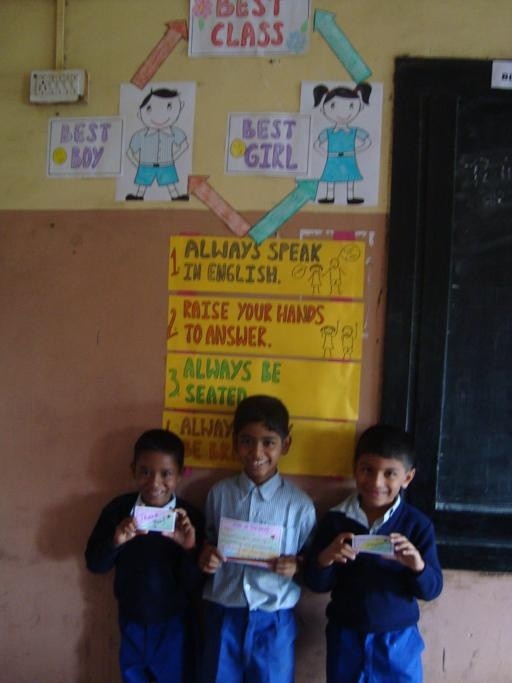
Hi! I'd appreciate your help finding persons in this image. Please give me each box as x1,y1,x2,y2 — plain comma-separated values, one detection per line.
84,427,205,682
199,394,318,683
297,423,445,682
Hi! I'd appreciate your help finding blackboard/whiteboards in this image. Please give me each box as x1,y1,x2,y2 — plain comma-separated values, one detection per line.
376,51,512,576
